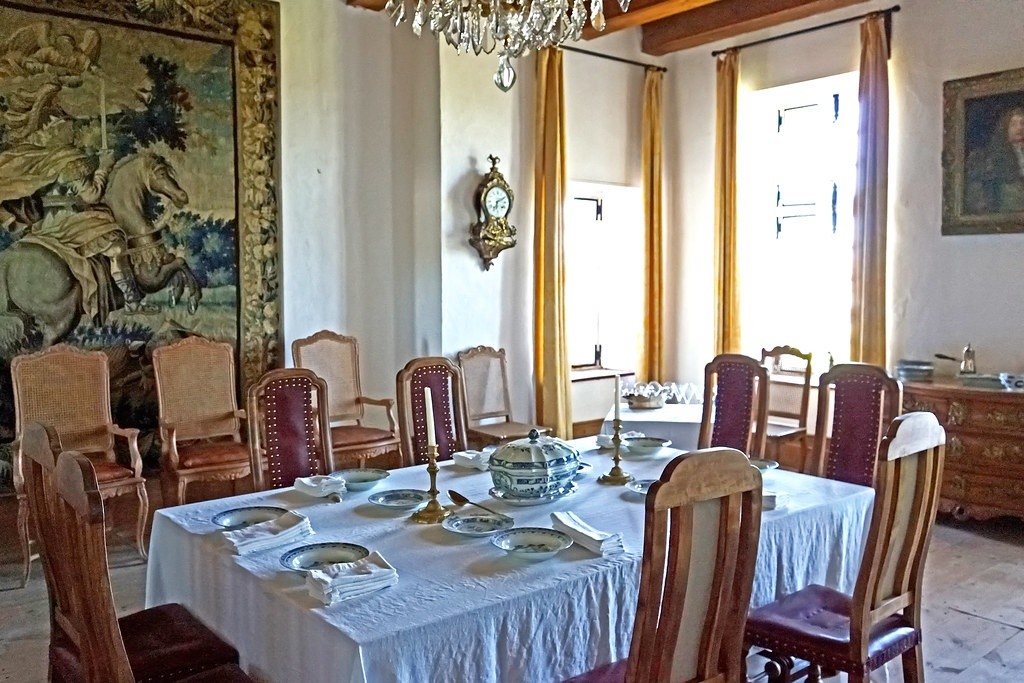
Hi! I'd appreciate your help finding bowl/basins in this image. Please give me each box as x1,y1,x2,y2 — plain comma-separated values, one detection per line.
999,372,1024,392
329,468,391,490
749,457,779,476
488,428,581,496
490,526,574,560
897,365,935,378
621,437,671,454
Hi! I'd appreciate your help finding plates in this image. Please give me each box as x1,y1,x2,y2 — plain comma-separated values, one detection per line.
368,489,429,510
441,512,515,537
489,481,578,507
626,478,656,494
279,542,369,577
211,506,287,530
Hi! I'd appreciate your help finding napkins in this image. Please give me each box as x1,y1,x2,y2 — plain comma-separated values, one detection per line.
223,509,315,555
549,511,627,557
453,448,491,471
596,431,643,450
304,550,398,604
763,489,788,509
293,474,347,498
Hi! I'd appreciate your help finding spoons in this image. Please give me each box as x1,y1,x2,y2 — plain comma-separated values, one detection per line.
446,489,497,514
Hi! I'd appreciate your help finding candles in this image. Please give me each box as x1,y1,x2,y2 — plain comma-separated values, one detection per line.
615,372,620,419
424,386,439,446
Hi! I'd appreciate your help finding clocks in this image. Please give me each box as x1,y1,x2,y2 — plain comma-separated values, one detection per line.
469,153,518,272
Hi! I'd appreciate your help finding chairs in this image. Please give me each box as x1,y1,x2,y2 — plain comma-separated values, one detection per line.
1,331,947,683
563,446,762,683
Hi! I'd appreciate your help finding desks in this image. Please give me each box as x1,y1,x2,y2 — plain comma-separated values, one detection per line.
889,372,1024,528
154,435,876,683
600,403,715,447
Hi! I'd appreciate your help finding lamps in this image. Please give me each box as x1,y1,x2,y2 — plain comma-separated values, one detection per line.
344,0,633,92
